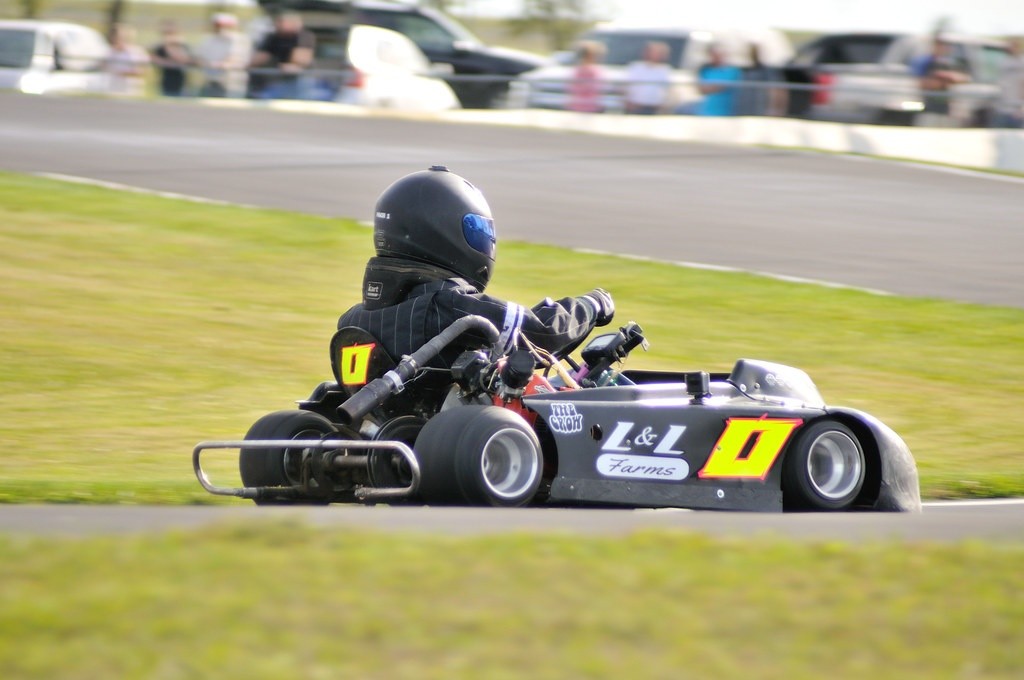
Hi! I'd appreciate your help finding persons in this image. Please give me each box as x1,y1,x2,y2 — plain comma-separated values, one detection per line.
97,25,147,95
918,35,969,127
327,167,614,423
696,41,788,117
148,21,193,95
624,42,675,114
251,12,316,92
189,14,250,98
567,43,606,112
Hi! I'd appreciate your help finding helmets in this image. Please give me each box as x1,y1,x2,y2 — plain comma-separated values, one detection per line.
373,166,496,294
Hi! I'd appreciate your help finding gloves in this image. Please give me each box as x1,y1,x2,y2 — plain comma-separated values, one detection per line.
585,288,614,327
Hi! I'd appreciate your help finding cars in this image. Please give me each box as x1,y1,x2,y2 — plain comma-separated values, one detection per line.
508,10,800,120
775,28,1023,131
0,19,144,98
242,0,545,114
